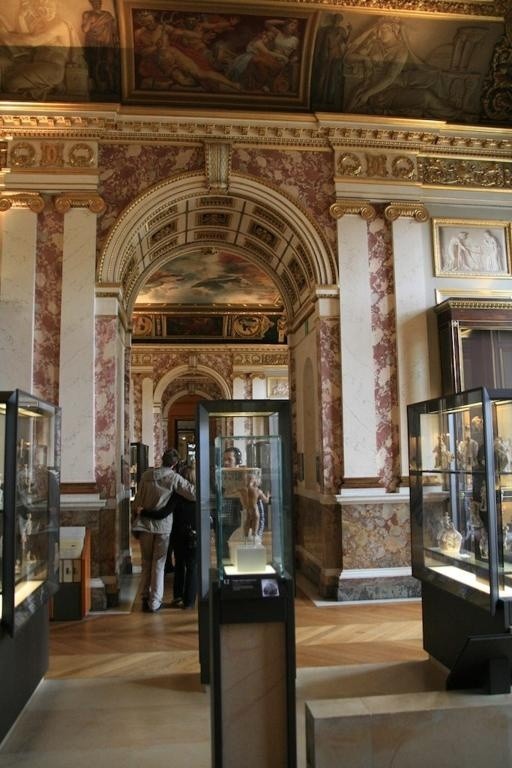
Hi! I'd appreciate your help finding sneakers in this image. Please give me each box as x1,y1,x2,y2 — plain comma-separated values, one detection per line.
143,598,195,612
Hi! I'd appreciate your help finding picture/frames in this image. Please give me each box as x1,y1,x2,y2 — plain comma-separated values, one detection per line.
112,1,322,110
431,217,511,280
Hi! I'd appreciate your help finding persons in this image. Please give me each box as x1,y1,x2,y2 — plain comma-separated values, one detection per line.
449,231,503,272
225,474,272,537
131,448,264,613
312,11,459,117
0,1,118,101
